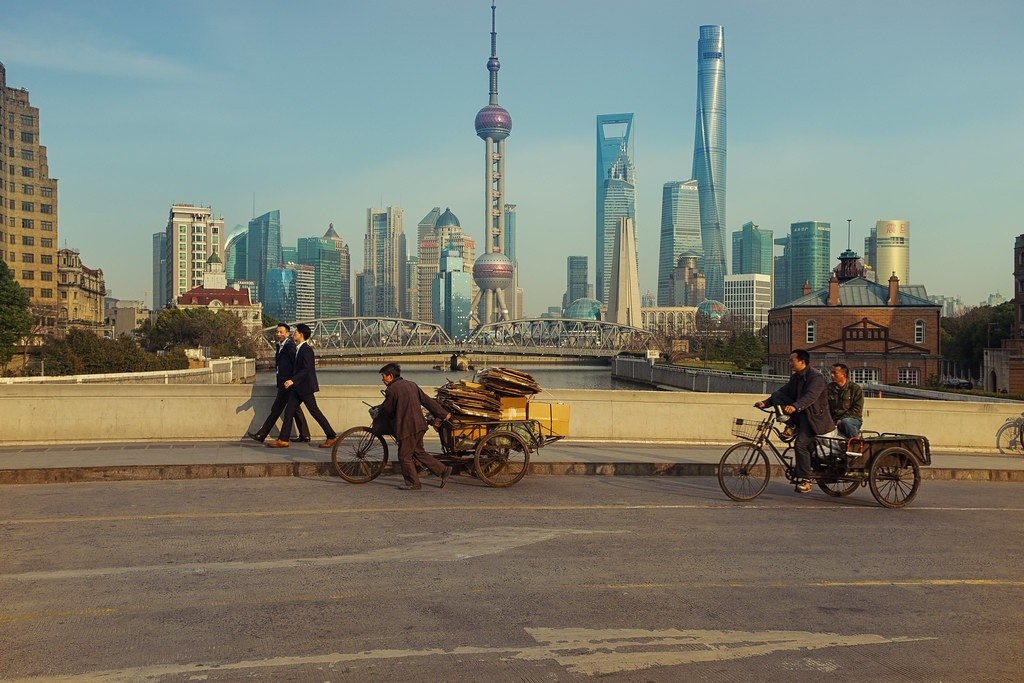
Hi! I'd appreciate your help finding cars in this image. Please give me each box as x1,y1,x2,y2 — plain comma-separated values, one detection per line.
950,379,973,391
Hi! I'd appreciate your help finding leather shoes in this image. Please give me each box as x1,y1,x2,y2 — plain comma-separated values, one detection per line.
248,431,264,442
320,437,339,448
267,439,289,448
290,434,310,442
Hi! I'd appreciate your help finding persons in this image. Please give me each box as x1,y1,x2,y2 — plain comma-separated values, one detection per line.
827,363,864,439
264,323,339,448
377,362,452,490
248,322,311,443
755,349,836,492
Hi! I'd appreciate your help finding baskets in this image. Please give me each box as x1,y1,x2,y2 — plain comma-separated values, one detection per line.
731,417,764,442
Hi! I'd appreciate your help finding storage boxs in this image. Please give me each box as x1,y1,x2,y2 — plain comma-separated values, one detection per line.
452,397,570,446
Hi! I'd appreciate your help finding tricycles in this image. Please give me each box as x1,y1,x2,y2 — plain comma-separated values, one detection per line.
717,402,932,509
332,390,566,489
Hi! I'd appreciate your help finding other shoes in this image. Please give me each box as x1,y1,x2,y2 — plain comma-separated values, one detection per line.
780,424,798,439
399,482,422,490
797,480,812,493
440,466,452,488
